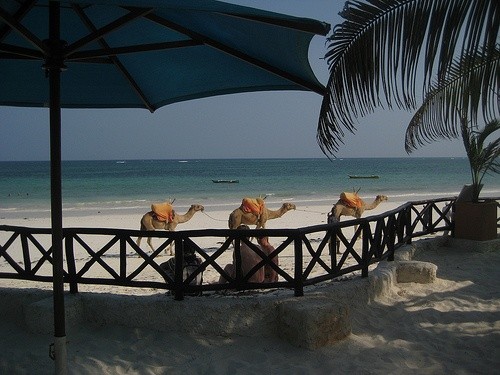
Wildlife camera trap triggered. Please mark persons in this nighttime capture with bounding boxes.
[328,208,342,254]
[218,224,277,283]
[161,240,202,295]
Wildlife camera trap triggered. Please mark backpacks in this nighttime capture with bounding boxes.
[182,260,204,296]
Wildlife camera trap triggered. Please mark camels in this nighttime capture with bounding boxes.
[228,202,296,245]
[333,194,388,238]
[135,203,204,255]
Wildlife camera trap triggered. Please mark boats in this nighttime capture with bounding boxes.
[210,180,240,184]
[346,175,378,179]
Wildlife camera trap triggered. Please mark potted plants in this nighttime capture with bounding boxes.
[454,111,500,241]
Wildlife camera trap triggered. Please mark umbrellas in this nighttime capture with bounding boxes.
[0,0,331,375]
